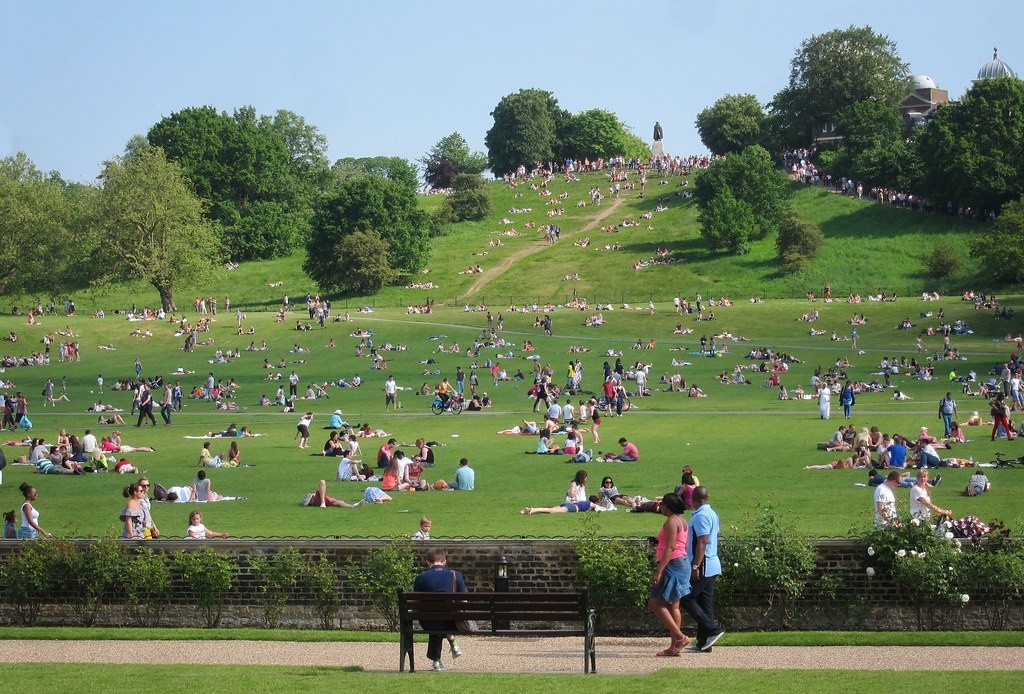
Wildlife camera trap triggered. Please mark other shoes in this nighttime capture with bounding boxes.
[149,447,157,452]
[74,468,86,475]
[966,482,975,496]
[934,473,941,486]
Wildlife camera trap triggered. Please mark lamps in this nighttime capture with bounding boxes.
[494,555,509,578]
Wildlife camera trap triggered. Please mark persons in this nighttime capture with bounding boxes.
[958,207,970,217]
[429,187,451,194]
[0,280,1024,540]
[646,493,690,656]
[414,548,465,670]
[784,148,930,212]
[653,122,663,141]
[990,210,995,224]
[225,261,239,271]
[680,485,724,652]
[457,154,726,281]
[948,200,952,215]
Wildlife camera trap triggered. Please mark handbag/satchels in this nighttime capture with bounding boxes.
[991,407,1005,415]
[18,414,32,428]
[450,569,478,632]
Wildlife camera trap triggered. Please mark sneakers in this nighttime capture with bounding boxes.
[701,631,724,651]
[684,645,703,653]
[433,660,445,672]
[452,647,462,660]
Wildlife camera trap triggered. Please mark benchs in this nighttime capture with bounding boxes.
[397,586,600,674]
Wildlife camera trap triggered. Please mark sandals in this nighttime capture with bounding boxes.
[657,648,681,656]
[671,635,690,655]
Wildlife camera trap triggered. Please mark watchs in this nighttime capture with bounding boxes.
[692,565,699,571]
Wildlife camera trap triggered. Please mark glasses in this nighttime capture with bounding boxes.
[137,490,143,493]
[140,485,150,488]
[860,445,866,447]
[605,481,612,484]
[416,459,421,463]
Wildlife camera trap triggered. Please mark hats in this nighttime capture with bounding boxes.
[334,410,342,415]
[920,426,929,431]
